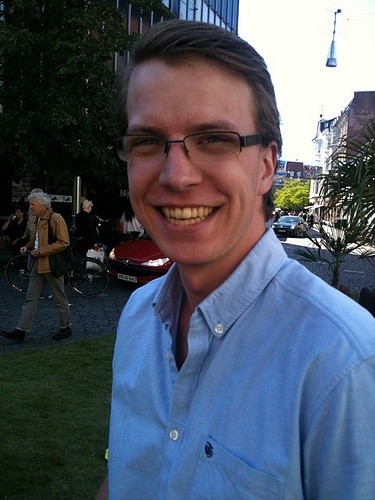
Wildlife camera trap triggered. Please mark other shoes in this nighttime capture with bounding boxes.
[51,327,72,341]
[0,329,25,342]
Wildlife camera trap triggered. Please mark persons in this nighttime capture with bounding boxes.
[1,207,28,275]
[13,188,46,280]
[0,192,72,342]
[96,18,375,499]
[71,199,100,268]
[119,202,145,239]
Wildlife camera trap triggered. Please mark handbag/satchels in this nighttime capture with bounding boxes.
[86,247,105,272]
[48,211,76,279]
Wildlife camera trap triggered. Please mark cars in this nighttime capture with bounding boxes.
[110,226,173,291]
[271,215,308,237]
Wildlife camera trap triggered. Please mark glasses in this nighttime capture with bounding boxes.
[116,131,265,163]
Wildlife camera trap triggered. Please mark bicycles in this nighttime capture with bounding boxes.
[5,253,108,297]
